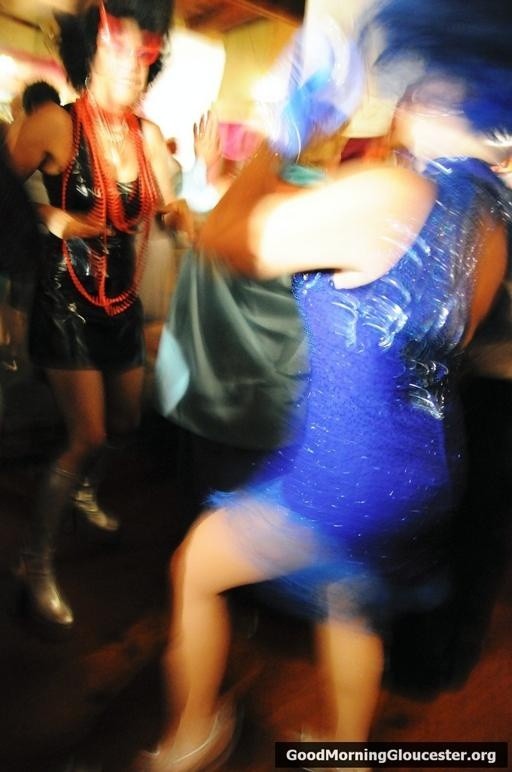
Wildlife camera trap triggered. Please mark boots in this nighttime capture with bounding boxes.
[70,431,128,534]
[13,465,77,630]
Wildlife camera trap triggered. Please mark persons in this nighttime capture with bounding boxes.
[0,0,512,772]
